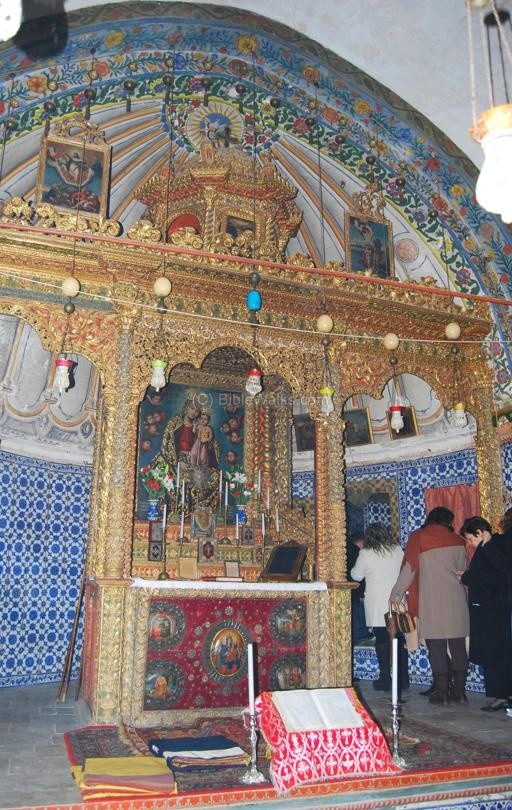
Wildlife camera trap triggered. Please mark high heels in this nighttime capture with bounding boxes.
[480,696,512,717]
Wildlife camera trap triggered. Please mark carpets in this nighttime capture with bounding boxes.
[63,715,512,809]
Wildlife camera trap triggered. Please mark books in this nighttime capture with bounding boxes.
[201,576,243,582]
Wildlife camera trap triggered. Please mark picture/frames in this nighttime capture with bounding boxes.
[35,135,111,221]
[224,559,241,578]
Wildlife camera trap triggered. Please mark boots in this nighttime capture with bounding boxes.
[418,670,468,706]
[398,649,409,689]
[373,643,390,691]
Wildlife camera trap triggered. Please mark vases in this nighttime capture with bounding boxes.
[146,499,160,522]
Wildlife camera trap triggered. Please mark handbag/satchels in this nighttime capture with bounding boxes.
[384,601,416,633]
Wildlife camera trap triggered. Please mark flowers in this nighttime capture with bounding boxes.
[140,462,175,500]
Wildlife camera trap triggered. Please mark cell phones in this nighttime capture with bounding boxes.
[450,569,463,577]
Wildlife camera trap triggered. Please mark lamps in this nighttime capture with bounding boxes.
[467,0,512,225]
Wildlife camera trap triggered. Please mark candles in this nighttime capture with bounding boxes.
[392,637,398,705]
[248,642,254,716]
[162,462,279,539]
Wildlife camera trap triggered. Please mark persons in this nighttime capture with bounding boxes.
[384,507,470,707]
[350,524,411,689]
[191,405,214,464]
[346,532,365,642]
[63,150,85,182]
[161,391,220,469]
[48,183,99,213]
[448,515,511,711]
